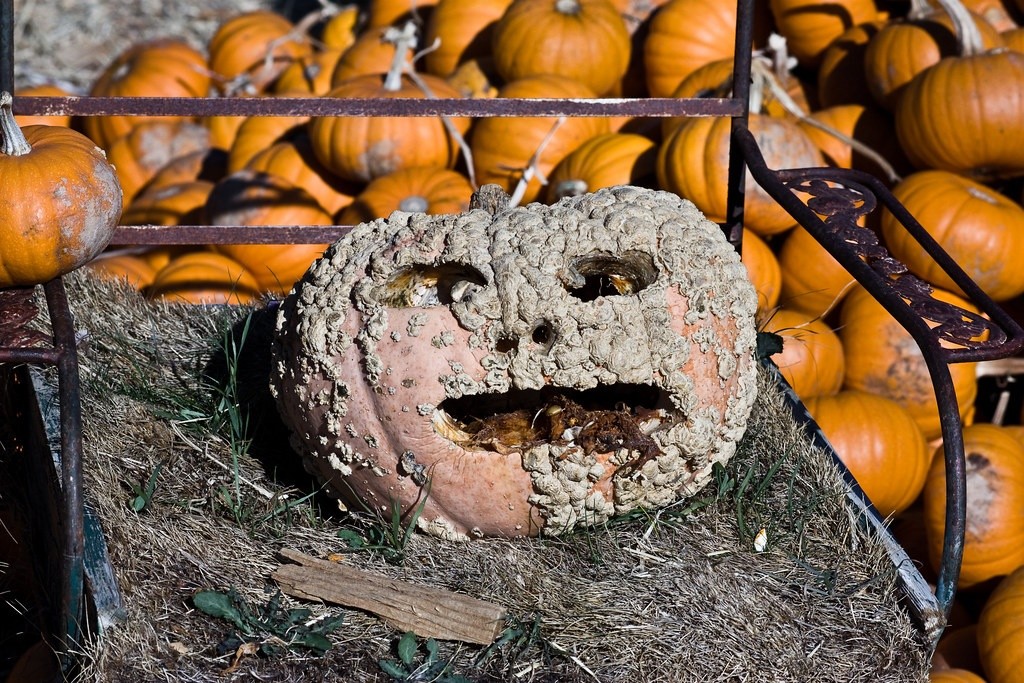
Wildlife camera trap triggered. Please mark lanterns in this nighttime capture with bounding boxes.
[262,182,760,541]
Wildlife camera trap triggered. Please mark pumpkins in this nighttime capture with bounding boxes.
[0,0,1024,683]
